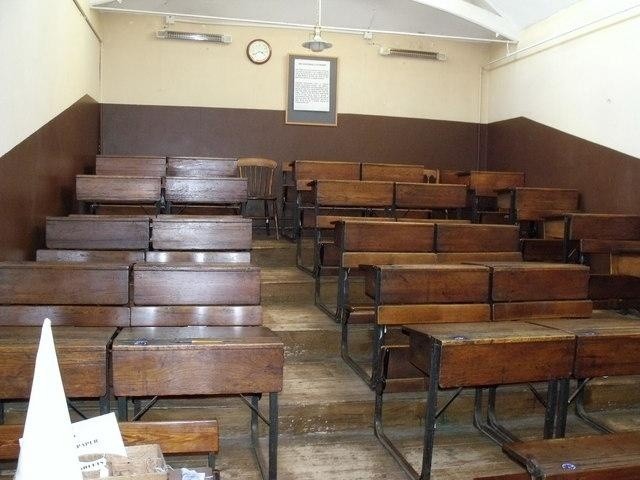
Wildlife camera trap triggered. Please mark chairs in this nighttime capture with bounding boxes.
[236,156,283,243]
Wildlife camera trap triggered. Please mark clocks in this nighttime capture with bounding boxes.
[247,38,272,64]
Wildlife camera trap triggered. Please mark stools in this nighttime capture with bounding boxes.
[0,154,290,477]
[289,156,637,471]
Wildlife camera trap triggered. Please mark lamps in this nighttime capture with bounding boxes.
[302,1,333,52]
